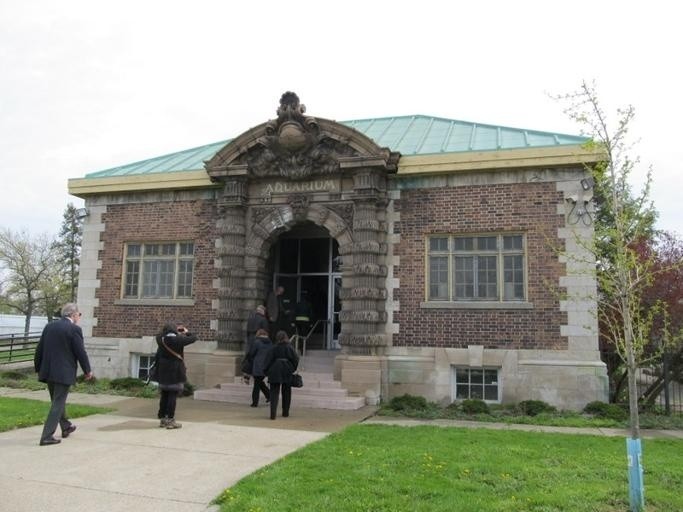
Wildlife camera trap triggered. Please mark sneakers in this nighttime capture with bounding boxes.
[250,397,271,407]
[166,419,181,429]
[271,412,288,419]
[160,418,166,426]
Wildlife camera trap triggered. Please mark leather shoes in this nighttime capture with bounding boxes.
[62,426,75,437]
[40,439,58,444]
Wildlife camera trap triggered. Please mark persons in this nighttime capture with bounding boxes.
[154,322,198,430]
[290,290,315,358]
[261,329,302,422]
[244,328,275,408]
[264,285,288,346]
[33,303,94,446]
[245,304,273,350]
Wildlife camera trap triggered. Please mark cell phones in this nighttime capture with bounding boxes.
[178,329,184,332]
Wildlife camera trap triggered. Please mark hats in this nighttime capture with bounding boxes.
[163,323,178,336]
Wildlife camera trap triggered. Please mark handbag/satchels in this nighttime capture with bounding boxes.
[148,359,159,381]
[291,375,302,387]
[240,356,253,373]
